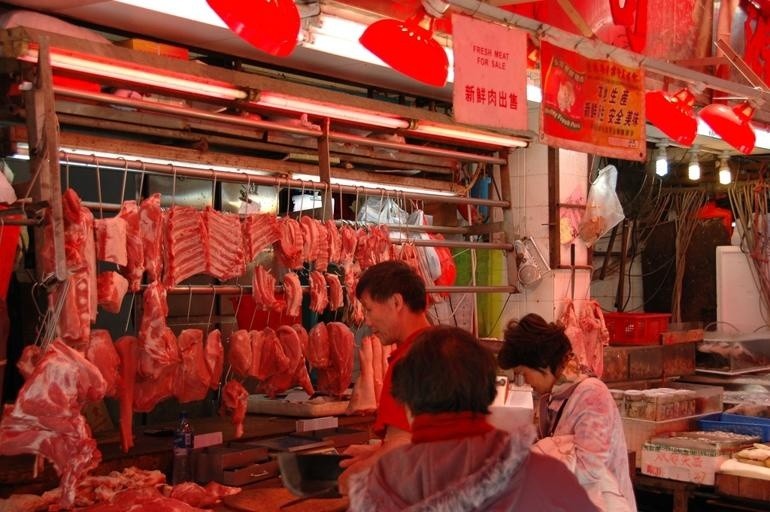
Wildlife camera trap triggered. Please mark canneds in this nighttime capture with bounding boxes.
[608,388,696,421]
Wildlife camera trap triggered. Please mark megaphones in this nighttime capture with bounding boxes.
[502,237,551,288]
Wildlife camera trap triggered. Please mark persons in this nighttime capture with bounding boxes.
[493,310,640,511]
[337,258,437,502]
[345,322,602,512]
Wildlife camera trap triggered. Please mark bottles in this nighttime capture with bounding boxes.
[171,411,195,485]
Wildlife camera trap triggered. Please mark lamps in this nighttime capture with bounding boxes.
[644,77,767,185]
[207,1,450,88]
